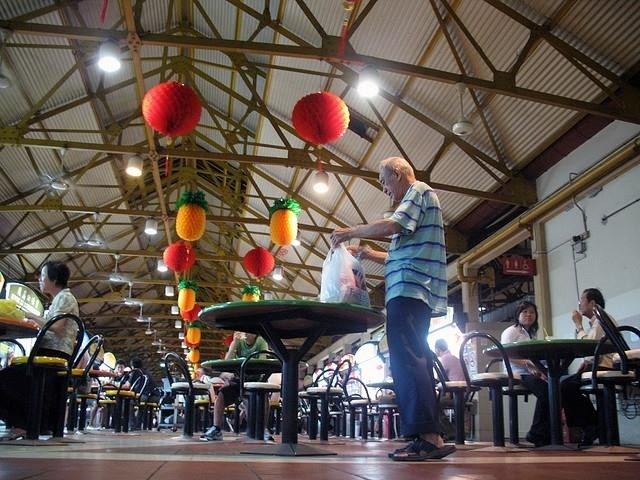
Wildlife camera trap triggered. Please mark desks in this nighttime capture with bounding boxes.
[481,338,617,454]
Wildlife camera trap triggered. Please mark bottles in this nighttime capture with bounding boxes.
[354,250,363,262]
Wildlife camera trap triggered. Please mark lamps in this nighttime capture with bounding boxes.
[312,170,328,195]
[125,152,145,177]
[293,230,301,248]
[156,256,197,379]
[98,37,122,74]
[356,63,380,98]
[272,263,284,281]
[144,217,159,236]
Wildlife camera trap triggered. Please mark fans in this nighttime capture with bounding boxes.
[0,27,17,89]
[37,147,71,190]
[78,212,169,361]
[452,82,473,137]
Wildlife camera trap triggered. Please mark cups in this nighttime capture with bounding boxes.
[112,381,120,390]
[545,336,556,341]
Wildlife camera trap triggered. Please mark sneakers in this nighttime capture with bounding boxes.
[525,433,551,446]
[577,427,598,445]
[1,428,26,440]
[87,426,99,431]
[263,430,276,443]
[199,426,223,441]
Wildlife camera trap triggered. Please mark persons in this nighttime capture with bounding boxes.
[558,287,627,451]
[435,340,467,403]
[329,157,456,461]
[200,328,276,442]
[67,333,177,437]
[0,262,79,442]
[501,301,560,448]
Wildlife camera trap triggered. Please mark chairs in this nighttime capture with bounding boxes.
[1,300,402,442]
[428,349,480,445]
[459,334,535,450]
[485,357,528,443]
[591,304,639,374]
[580,326,640,453]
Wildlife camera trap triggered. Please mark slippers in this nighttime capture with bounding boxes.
[388,439,456,461]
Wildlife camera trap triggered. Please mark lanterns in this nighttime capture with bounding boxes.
[162,240,197,273]
[175,190,207,260]
[242,247,274,279]
[270,196,301,246]
[143,80,202,178]
[177,281,261,378]
[292,93,350,175]
